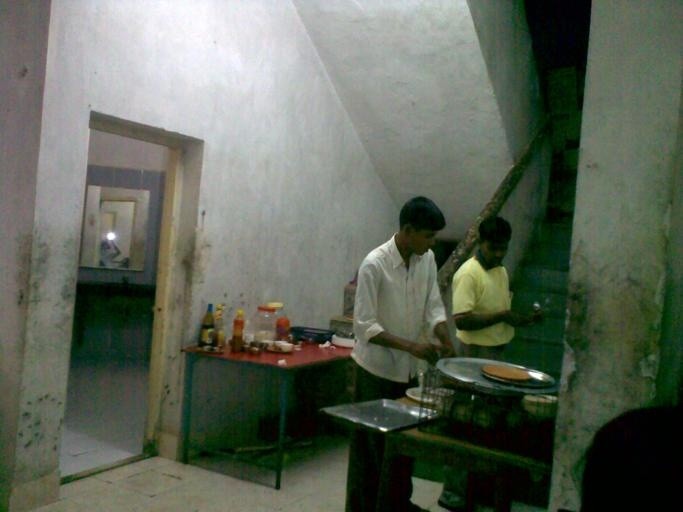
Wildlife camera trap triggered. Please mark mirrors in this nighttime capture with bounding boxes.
[78,183,151,273]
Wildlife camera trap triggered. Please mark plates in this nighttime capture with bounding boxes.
[405,384,446,406]
[479,364,533,382]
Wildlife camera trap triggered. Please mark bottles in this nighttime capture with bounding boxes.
[196,301,290,354]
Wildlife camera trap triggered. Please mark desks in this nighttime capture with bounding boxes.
[334,397,552,512]
[180,342,354,489]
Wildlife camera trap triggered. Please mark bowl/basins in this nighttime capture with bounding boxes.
[522,394,559,419]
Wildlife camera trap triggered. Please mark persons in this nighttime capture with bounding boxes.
[438,216,536,512]
[343,195,454,512]
[100,239,121,266]
